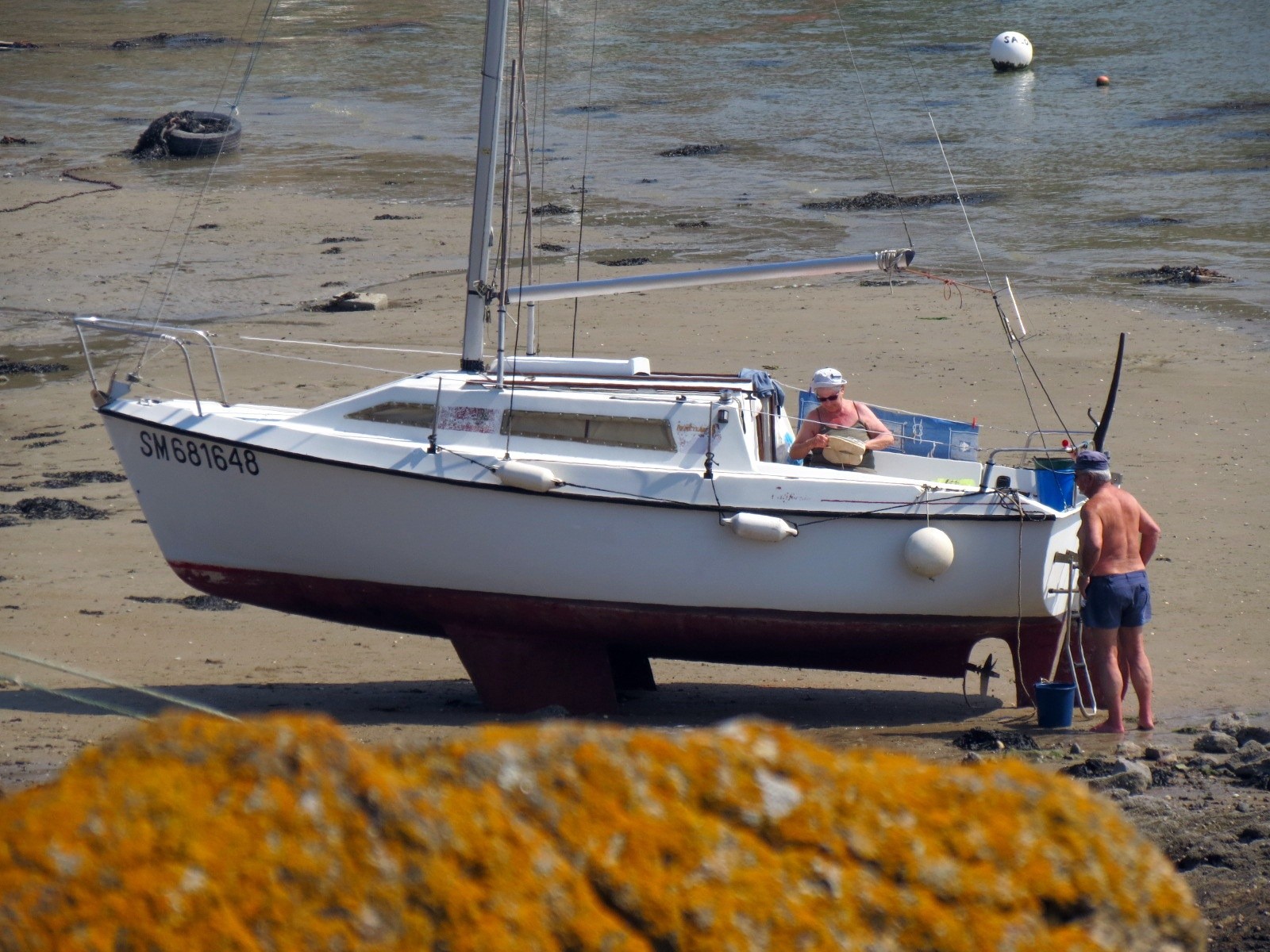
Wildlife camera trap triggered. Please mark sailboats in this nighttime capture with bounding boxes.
[75,0,1131,716]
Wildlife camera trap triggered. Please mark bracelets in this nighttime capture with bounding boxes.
[805,438,815,451]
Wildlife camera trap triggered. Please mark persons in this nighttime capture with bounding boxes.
[789,368,894,474]
[1075,451,1161,734]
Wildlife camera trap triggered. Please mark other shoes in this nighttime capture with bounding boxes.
[822,447,863,466]
[826,434,866,455]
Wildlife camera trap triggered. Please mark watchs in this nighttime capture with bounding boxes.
[1079,571,1087,578]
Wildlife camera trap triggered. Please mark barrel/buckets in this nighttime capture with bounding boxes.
[1032,456,1076,475]
[1034,469,1076,511]
[1034,680,1077,729]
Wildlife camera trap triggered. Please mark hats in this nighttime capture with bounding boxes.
[1071,451,1109,470]
[809,368,847,388]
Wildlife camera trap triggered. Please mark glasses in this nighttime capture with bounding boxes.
[816,389,841,403]
